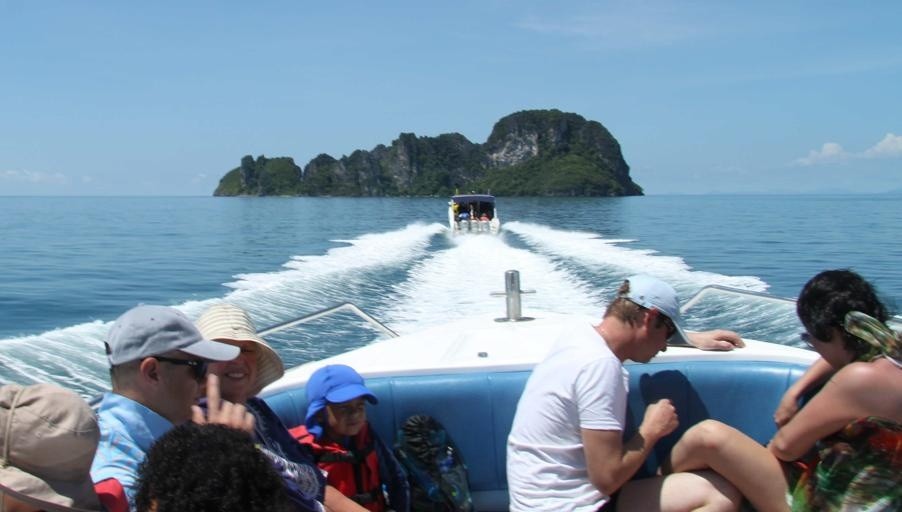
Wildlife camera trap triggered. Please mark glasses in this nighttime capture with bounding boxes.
[153,354,209,383]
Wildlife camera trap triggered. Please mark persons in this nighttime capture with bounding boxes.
[0,307,394,511]
[506,275,739,511]
[452,202,490,222]
[659,270,902,512]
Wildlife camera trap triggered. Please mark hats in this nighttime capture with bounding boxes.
[193,304,285,395]
[302,363,378,426]
[615,275,696,349]
[0,384,100,512]
[105,304,241,364]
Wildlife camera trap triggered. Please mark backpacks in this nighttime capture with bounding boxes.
[393,413,473,510]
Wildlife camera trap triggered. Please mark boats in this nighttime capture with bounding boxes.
[447,188,500,237]
[76,285,902,510]
